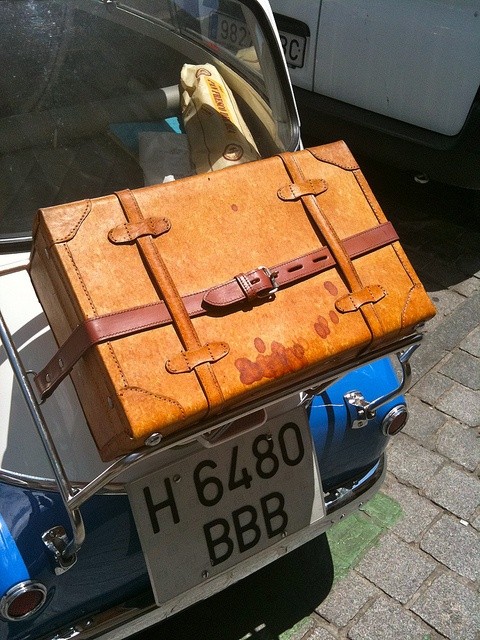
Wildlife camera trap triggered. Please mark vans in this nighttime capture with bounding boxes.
[2,0,421,638]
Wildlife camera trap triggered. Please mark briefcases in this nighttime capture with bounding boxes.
[26,139,436,462]
